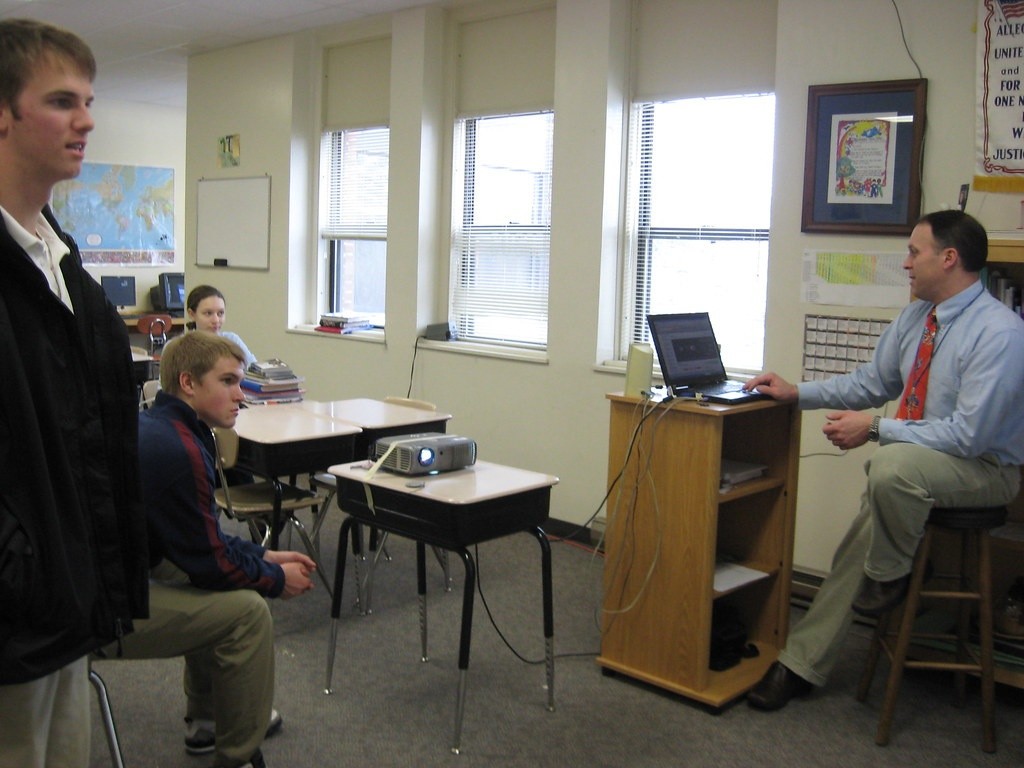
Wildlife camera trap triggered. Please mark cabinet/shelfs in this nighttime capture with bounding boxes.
[957,242,1024,707]
[600,388,809,707]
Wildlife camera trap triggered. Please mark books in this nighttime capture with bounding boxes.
[314,312,373,334]
[978,267,1024,320]
[240,359,306,407]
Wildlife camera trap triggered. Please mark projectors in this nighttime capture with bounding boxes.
[374,432,477,475]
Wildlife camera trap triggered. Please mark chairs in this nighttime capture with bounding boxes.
[130,345,148,354]
[206,423,335,613]
[137,314,173,378]
[143,378,162,406]
[376,399,434,575]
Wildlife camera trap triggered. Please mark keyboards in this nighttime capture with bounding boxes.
[120,315,141,320]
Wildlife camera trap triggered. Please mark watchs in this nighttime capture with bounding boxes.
[867,416,881,443]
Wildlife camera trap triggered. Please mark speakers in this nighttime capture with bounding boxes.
[624,343,654,399]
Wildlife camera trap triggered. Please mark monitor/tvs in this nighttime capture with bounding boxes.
[100,276,136,309]
[159,272,185,310]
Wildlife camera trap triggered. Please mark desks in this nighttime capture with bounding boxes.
[301,395,452,616]
[227,401,365,618]
[320,458,572,754]
[131,350,154,388]
[119,311,185,333]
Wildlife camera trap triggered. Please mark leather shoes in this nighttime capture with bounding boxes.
[852,572,912,620]
[746,660,821,712]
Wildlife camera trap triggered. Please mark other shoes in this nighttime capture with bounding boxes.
[184,708,282,753]
[213,747,266,768]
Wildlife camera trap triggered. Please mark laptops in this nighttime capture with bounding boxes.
[648,312,773,405]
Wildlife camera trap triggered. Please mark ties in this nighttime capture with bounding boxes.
[893,304,939,421]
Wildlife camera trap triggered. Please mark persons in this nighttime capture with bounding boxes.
[741,211,1024,712]
[0,19,148,768]
[186,285,257,366]
[88,330,317,768]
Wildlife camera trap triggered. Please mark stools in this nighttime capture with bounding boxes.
[844,505,1007,755]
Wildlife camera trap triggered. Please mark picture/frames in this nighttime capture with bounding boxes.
[799,77,928,235]
